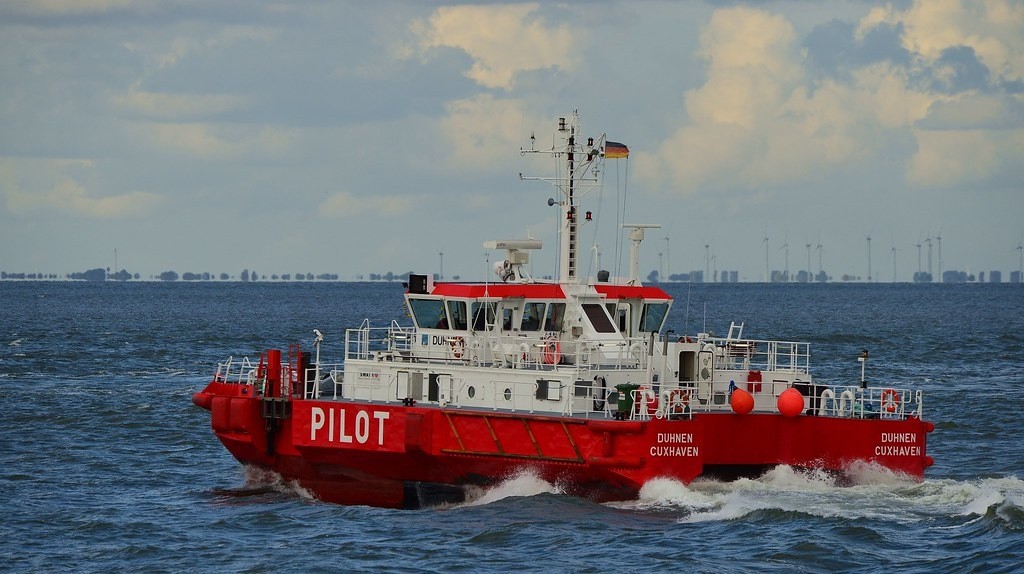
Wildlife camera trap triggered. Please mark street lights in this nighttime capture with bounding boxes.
[662,228,944,283]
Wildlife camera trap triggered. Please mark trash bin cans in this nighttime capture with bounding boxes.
[614,384,640,417]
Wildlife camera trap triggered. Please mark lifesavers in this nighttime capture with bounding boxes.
[452,336,464,358]
[669,389,687,411]
[678,337,693,343]
[883,390,899,412]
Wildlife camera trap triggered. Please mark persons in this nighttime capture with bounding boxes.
[436,306,450,329]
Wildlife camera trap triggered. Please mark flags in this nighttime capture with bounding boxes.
[604,140,630,158]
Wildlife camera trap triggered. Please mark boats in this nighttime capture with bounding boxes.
[193,108,936,507]
